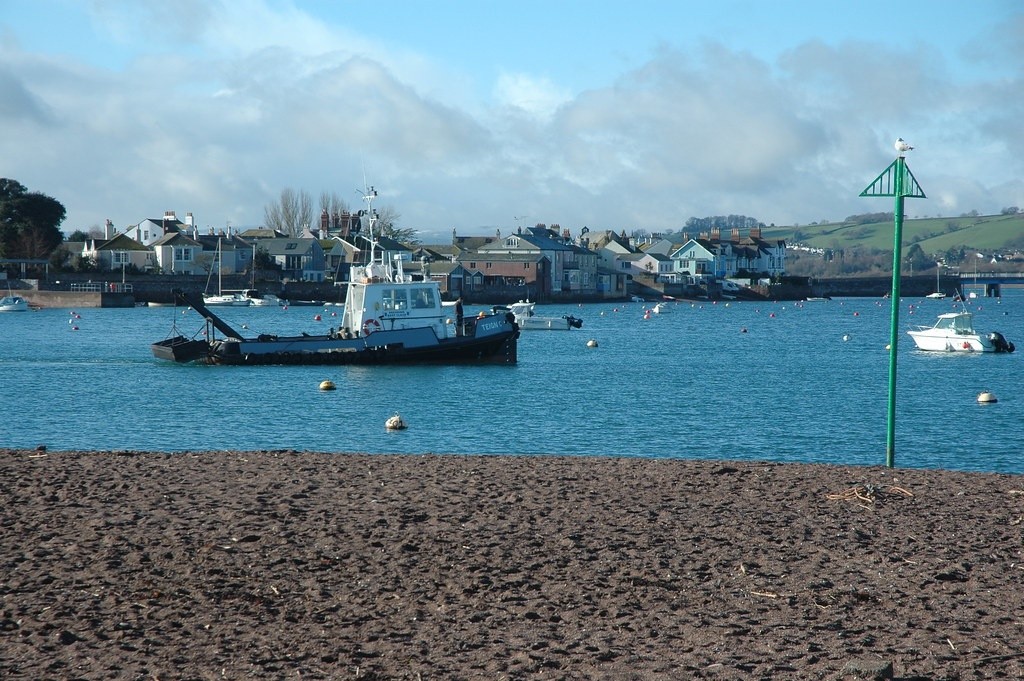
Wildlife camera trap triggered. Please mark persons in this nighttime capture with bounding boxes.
[454,297,464,337]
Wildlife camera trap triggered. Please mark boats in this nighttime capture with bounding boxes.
[806,296,830,302]
[0,295,29,311]
[925,292,947,300]
[906,287,1015,358]
[202,237,327,306]
[151,151,519,364]
[505,297,583,330]
